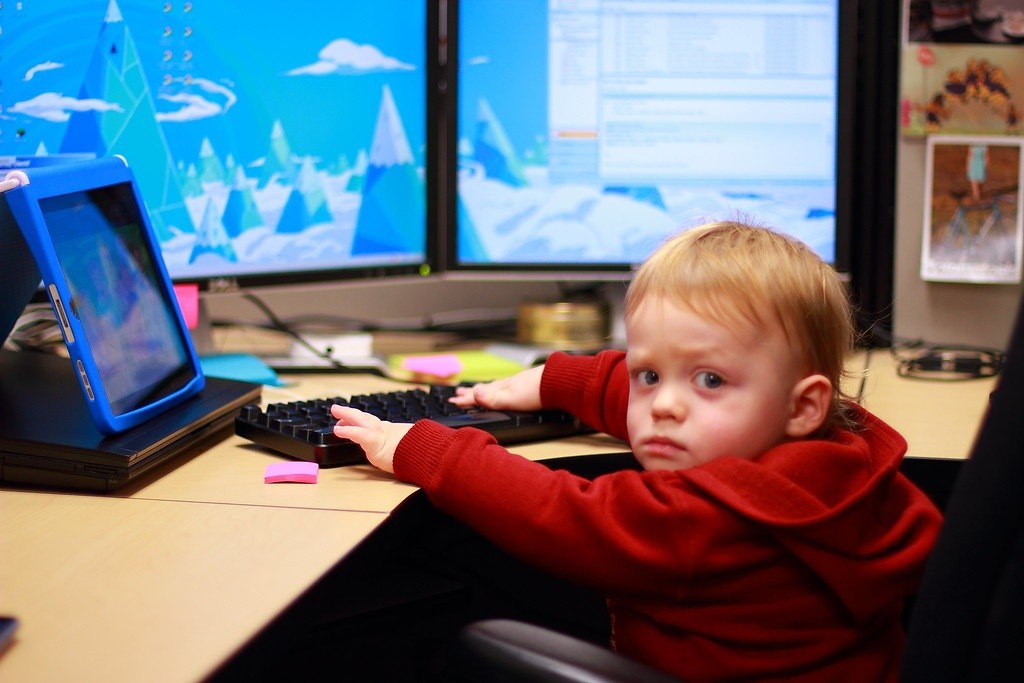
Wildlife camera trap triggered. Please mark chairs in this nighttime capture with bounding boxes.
[462,296,1024,683]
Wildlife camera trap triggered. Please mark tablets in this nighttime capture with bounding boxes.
[5,154,206,434]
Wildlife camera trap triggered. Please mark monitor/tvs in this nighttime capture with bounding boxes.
[0,0,857,357]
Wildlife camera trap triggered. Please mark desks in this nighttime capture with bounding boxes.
[0,491,390,683]
[858,350,1000,478]
[128,340,871,516]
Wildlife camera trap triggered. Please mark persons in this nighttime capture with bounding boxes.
[327,221,943,683]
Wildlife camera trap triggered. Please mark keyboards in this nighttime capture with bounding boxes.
[234,379,603,468]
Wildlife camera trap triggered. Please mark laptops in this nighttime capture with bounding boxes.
[0,349,262,481]
